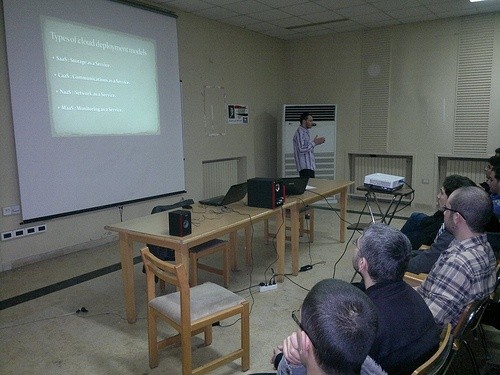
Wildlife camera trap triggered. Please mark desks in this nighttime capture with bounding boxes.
[104,178,355,324]
[357,185,416,225]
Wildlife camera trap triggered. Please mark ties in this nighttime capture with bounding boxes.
[434,222,445,244]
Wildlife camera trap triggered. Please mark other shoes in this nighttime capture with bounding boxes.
[305,214,310,219]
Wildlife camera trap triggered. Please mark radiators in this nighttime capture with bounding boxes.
[202,159,238,200]
[446,160,489,184]
[353,157,406,201]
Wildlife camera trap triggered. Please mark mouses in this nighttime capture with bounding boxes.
[182,205,192,209]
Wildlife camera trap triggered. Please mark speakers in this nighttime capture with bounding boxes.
[168,209,192,237]
[247,176,286,209]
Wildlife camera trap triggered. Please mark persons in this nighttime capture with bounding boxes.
[397,147,500,264]
[292,113,325,219]
[399,176,494,275]
[244,222,443,375]
[351,186,497,341]
[275,278,389,375]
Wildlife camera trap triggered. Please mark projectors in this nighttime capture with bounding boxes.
[364,173,405,188]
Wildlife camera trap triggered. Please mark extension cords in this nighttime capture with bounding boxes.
[256,283,277,292]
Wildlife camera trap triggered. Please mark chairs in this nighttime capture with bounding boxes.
[264,205,315,243]
[139,247,250,375]
[403,244,500,375]
[151,199,231,289]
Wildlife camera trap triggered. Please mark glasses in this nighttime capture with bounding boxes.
[291,310,318,351]
[442,206,466,220]
[352,238,364,257]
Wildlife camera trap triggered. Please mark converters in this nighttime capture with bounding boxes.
[300,265,313,271]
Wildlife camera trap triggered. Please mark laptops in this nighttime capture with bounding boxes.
[199,182,248,206]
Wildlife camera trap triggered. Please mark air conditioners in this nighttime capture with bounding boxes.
[276,103,338,205]
